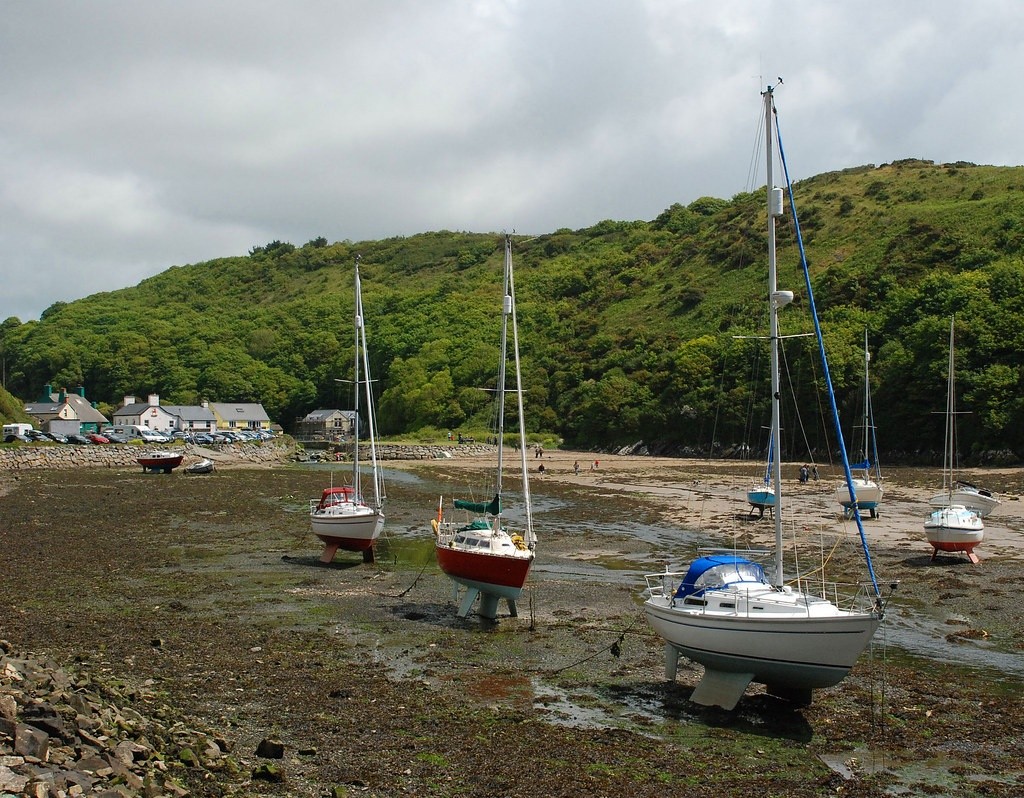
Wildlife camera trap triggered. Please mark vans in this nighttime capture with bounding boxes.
[114,424,156,441]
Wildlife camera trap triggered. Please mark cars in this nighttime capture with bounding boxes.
[151,429,277,444]
[5,430,128,445]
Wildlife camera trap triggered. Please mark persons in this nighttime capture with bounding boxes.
[539,463,545,475]
[573,462,580,474]
[595,460,598,467]
[514,443,518,453]
[493,436,498,447]
[447,431,455,442]
[535,446,543,459]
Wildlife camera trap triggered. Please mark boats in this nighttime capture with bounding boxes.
[136,455,184,468]
[186,460,216,473]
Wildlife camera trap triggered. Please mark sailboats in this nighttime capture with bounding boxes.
[921,316,1003,566]
[430,225,538,620]
[310,252,389,565]
[644,75,896,712]
[834,328,885,520]
[745,373,788,517]
